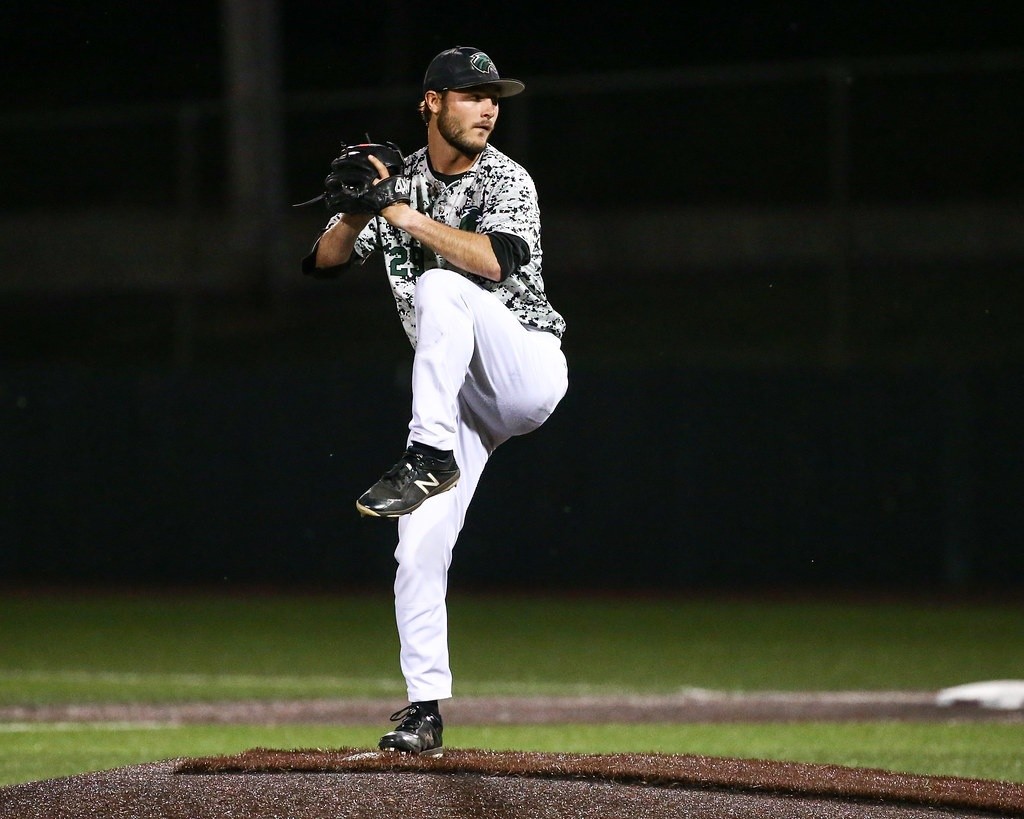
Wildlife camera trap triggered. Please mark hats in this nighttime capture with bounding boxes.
[424,45,525,97]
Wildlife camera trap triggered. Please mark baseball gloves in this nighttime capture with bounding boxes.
[290,132,412,217]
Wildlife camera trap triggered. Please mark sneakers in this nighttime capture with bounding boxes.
[379,704,442,760]
[355,447,460,517]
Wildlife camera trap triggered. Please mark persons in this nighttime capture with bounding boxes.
[301,42,568,756]
